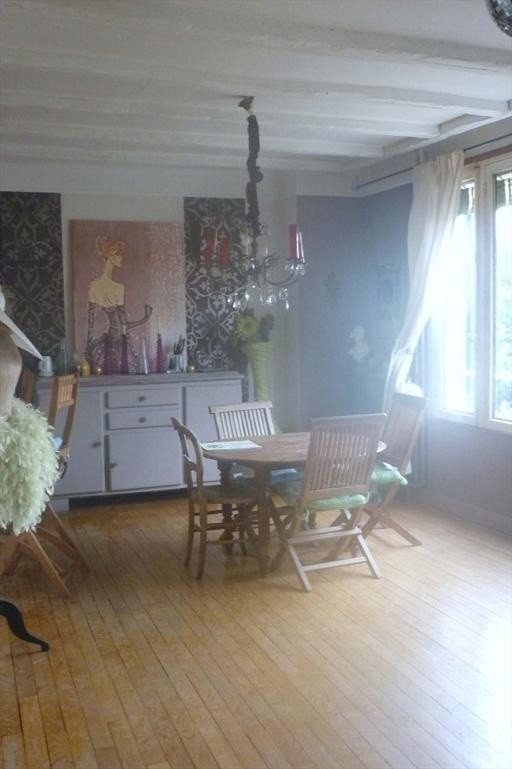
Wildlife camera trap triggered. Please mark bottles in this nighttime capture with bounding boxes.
[137,338,149,374]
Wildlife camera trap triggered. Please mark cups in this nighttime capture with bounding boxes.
[36,355,55,377]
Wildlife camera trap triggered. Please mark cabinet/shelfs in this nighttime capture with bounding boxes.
[31,369,244,516]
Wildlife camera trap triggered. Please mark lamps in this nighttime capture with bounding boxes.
[205,97,308,313]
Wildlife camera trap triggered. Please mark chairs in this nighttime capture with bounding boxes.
[0,373,91,600]
[172,392,428,592]
